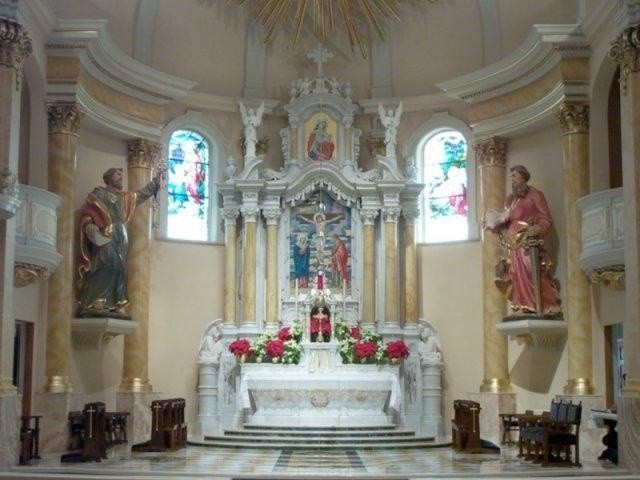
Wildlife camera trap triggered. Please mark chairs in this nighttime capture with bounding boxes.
[498,397,583,470]
[20,410,131,461]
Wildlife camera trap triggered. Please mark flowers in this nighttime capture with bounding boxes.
[227,317,412,372]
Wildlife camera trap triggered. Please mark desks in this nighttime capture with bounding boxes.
[584,407,618,467]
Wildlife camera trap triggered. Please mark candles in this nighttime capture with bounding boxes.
[295,277,299,317]
[343,277,346,317]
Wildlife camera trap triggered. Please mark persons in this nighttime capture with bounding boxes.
[199,326,223,361]
[77,164,165,317]
[294,76,353,99]
[381,108,401,150]
[294,233,312,286]
[243,108,260,152]
[308,117,334,162]
[419,327,442,362]
[329,235,350,290]
[298,211,341,270]
[481,166,560,311]
